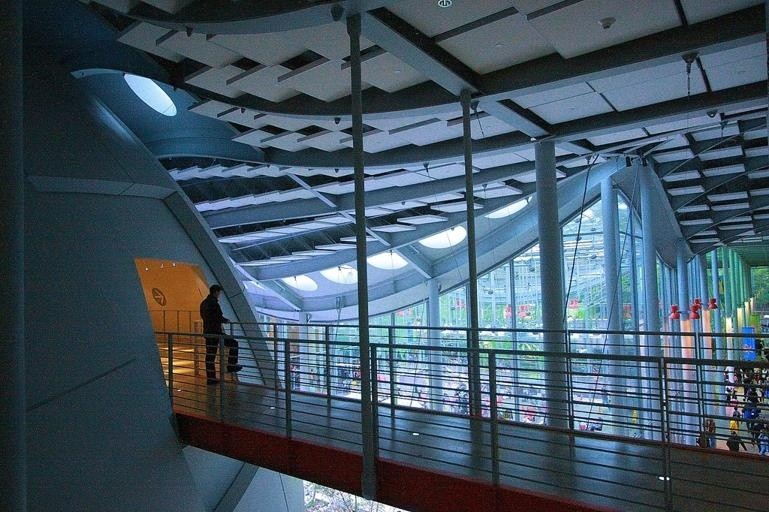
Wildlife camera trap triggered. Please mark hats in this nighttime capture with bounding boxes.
[210,285,223,292]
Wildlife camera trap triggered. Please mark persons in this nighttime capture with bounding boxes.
[288,364,610,431]
[200,286,243,384]
[694,366,769,455]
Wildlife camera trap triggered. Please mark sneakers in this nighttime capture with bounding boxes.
[207,379,220,384]
[227,366,242,372]
